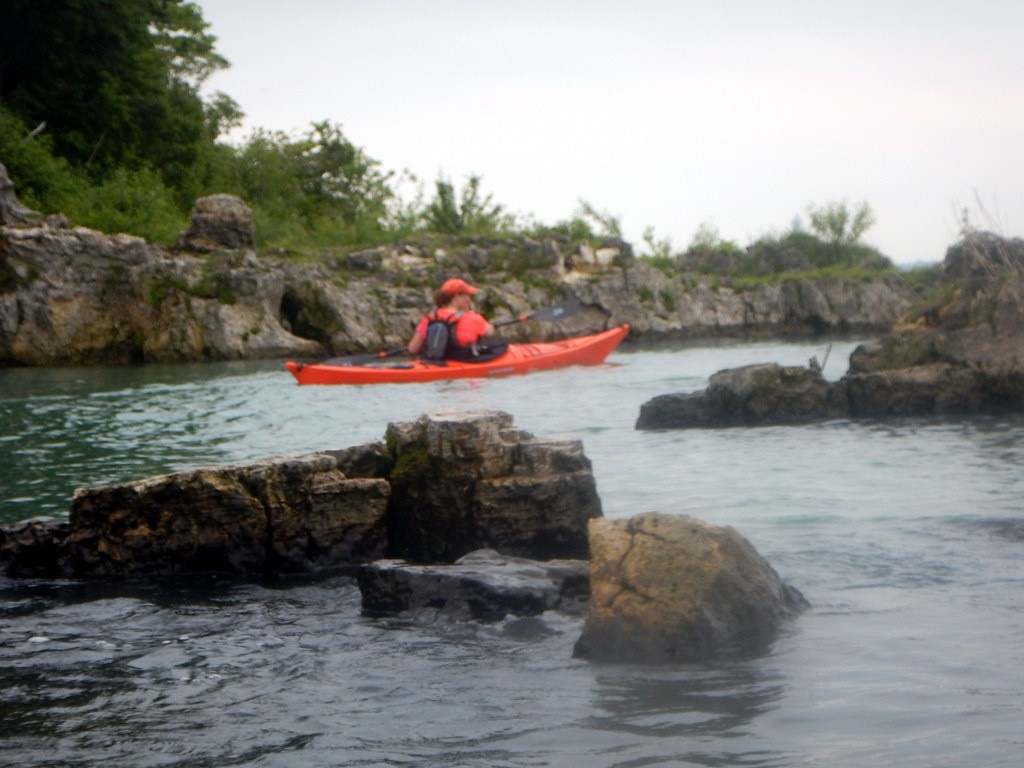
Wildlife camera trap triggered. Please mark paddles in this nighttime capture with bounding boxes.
[323,298,582,367]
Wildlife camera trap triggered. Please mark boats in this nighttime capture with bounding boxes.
[283,324,632,384]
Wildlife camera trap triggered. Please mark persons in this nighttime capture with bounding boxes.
[408,278,498,360]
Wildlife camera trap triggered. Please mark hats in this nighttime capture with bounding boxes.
[441,278,477,294]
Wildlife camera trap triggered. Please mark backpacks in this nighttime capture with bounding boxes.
[423,307,464,360]
